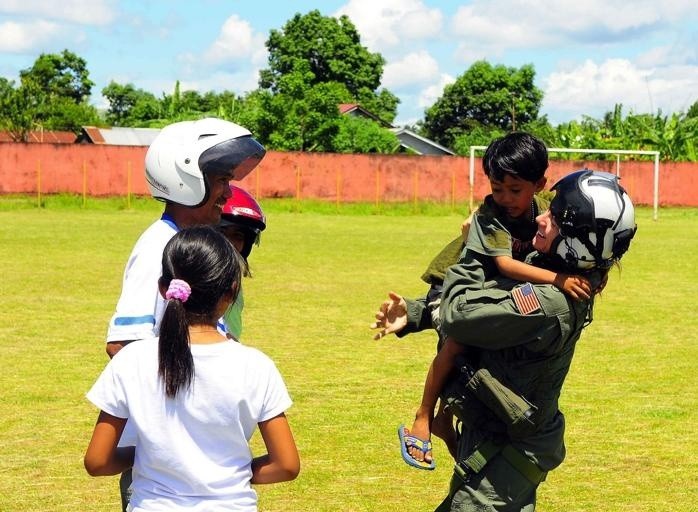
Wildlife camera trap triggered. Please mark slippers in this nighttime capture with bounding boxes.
[398,424,436,470]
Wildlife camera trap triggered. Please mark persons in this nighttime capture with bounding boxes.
[397,131,592,472]
[105,115,268,511]
[371,170,637,512]
[214,183,267,340]
[83,224,300,511]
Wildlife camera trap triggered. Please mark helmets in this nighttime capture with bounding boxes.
[145,117,266,235]
[549,169,637,272]
